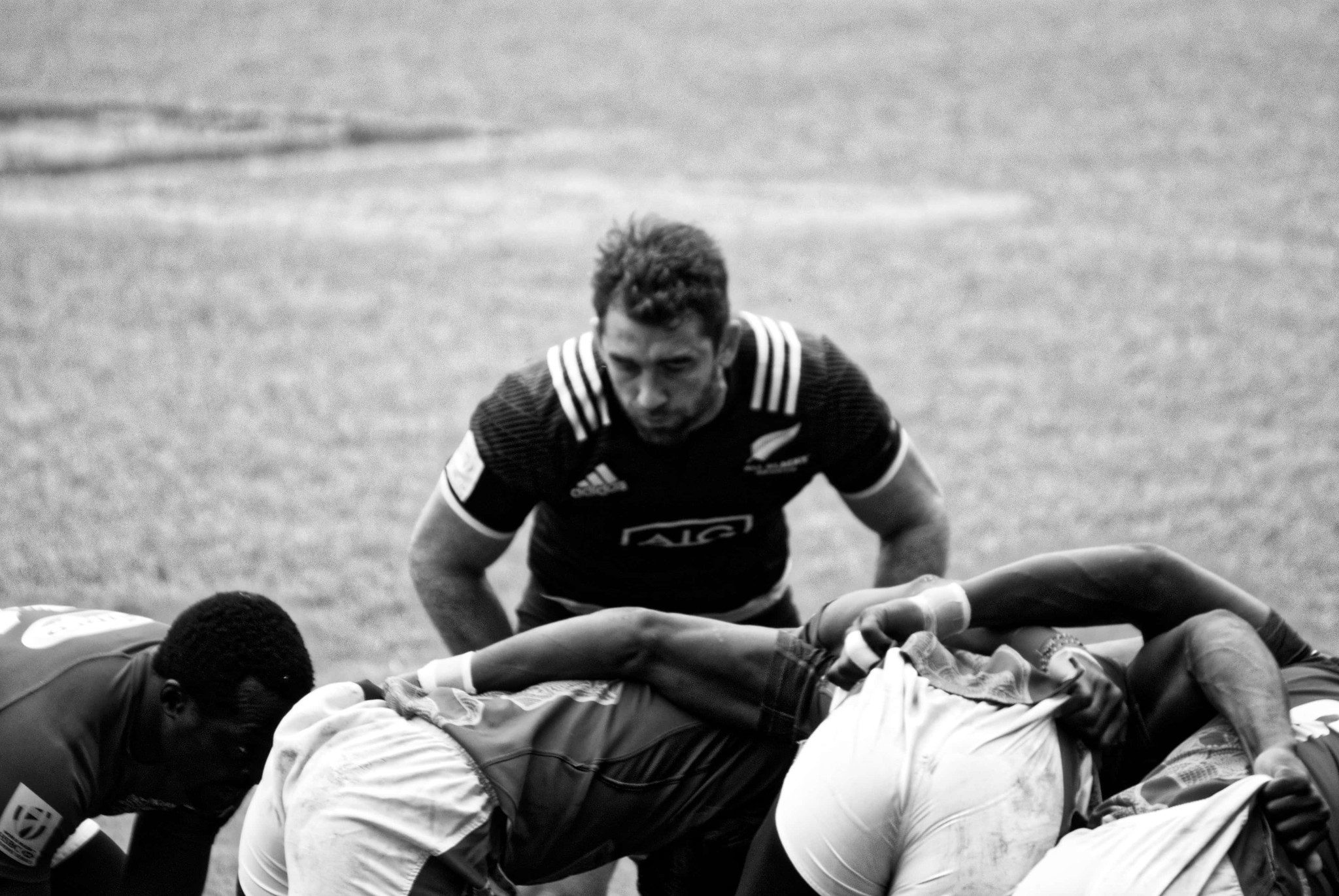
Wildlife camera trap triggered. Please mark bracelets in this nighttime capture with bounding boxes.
[911,581,971,639]
[416,650,476,695]
[1039,633,1083,672]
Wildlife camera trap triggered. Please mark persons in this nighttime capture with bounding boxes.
[407,216,951,896]
[239,544,1339,896]
[0,590,315,896]
